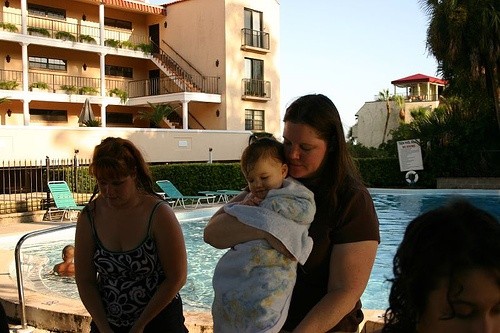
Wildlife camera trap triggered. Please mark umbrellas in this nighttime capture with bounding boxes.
[78,98,95,124]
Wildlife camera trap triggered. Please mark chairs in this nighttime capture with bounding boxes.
[46,181,85,221]
[156,177,244,210]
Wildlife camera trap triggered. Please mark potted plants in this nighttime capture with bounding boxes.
[0,80,129,103]
[0,21,152,56]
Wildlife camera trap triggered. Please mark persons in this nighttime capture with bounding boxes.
[203,93,381,333]
[74,136,188,333]
[382,198,500,332]
[212,138,314,332]
[53,245,77,273]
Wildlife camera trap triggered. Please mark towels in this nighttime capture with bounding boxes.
[211,178,316,333]
